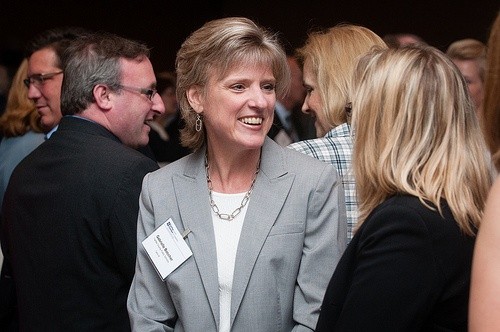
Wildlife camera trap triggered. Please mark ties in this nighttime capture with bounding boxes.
[286,116,296,140]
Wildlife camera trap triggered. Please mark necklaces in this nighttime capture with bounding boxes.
[203,150,261,220]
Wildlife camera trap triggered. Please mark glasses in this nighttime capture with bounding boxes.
[345,102,352,117]
[23,71,65,89]
[91,81,157,103]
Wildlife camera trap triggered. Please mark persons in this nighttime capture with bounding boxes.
[127,16,347,332]
[133,74,194,168]
[22,28,98,142]
[313,38,500,332]
[468,16,500,332]
[265,50,308,148]
[286,25,390,247]
[1,59,45,201]
[1,30,178,332]
[443,39,486,130]
